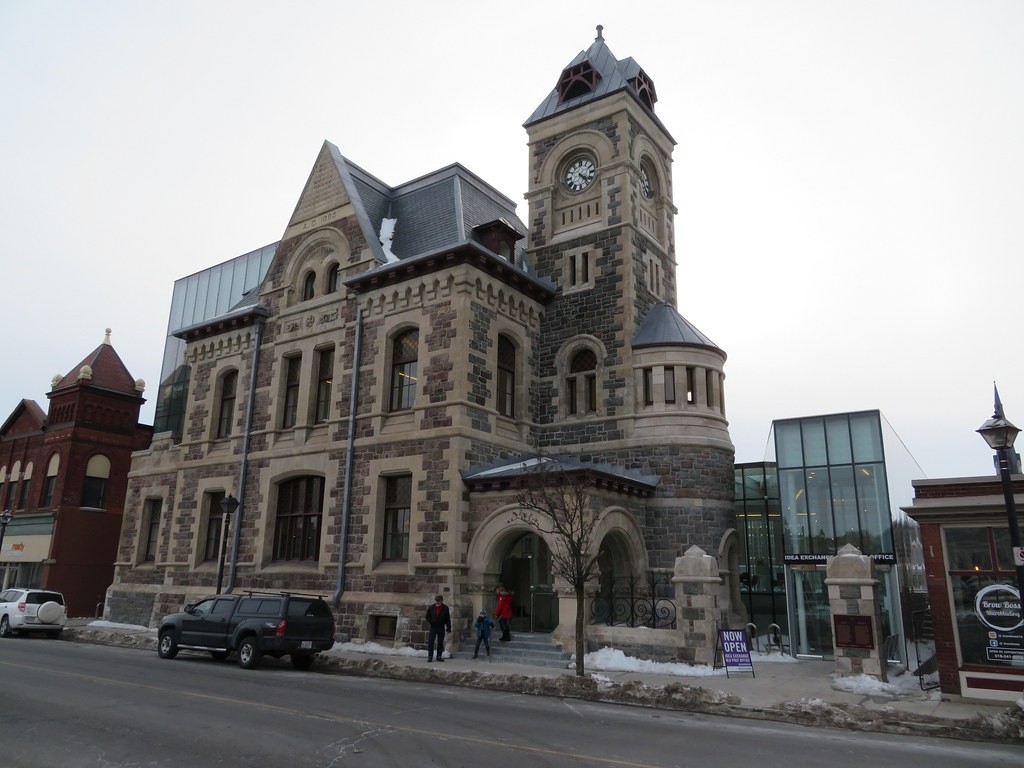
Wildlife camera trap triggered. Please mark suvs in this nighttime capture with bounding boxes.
[0,588,68,640]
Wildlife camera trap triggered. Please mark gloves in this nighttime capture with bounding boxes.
[489,622,494,629]
[446,626,451,633]
[478,617,482,622]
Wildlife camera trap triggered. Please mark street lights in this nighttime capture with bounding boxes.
[216,492,242,594]
[973,380,1024,622]
[0,508,16,552]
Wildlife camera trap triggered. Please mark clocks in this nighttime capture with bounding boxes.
[566,159,595,190]
[640,167,649,197]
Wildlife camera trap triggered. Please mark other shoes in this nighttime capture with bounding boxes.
[499,633,511,642]
[472,652,479,659]
[427,656,433,662]
[485,652,490,656]
[436,656,444,662]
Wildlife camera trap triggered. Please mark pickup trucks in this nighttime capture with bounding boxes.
[156,589,339,669]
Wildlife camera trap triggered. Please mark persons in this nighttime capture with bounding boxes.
[472,609,494,659]
[494,586,514,641]
[425,595,451,662]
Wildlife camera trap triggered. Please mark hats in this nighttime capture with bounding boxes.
[480,609,487,615]
[493,582,505,591]
[434,596,443,601]
